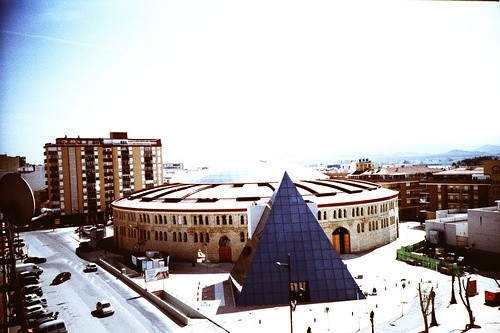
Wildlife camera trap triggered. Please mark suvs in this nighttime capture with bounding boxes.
[97,300,115,316]
[86,263,98,271]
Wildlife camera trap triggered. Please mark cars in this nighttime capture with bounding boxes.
[53,271,71,285]
[4,233,67,333]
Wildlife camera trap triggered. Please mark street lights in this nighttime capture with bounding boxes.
[276,253,294,333]
[431,291,438,326]
[450,269,457,304]
[370,311,374,333]
